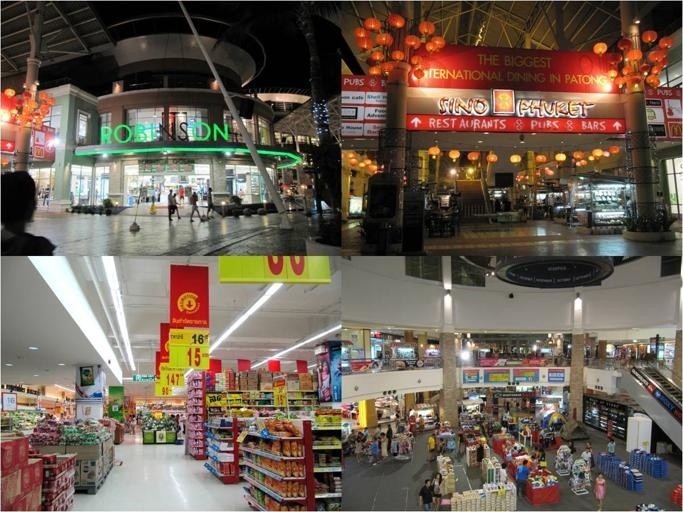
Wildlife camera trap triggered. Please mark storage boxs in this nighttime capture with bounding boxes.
[0,434,77,512]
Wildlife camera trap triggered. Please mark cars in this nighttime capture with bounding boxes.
[545,190,628,227]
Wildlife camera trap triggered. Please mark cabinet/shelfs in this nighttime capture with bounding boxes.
[583,395,628,441]
[0,407,42,436]
[32,436,114,494]
[113,429,124,444]
[142,427,177,444]
[185,370,343,512]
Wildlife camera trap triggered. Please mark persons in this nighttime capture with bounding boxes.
[347,410,616,511]
[167,185,215,222]
[42,184,49,205]
[1,170,57,256]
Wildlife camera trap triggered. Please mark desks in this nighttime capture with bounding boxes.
[518,431,533,447]
[493,434,560,506]
